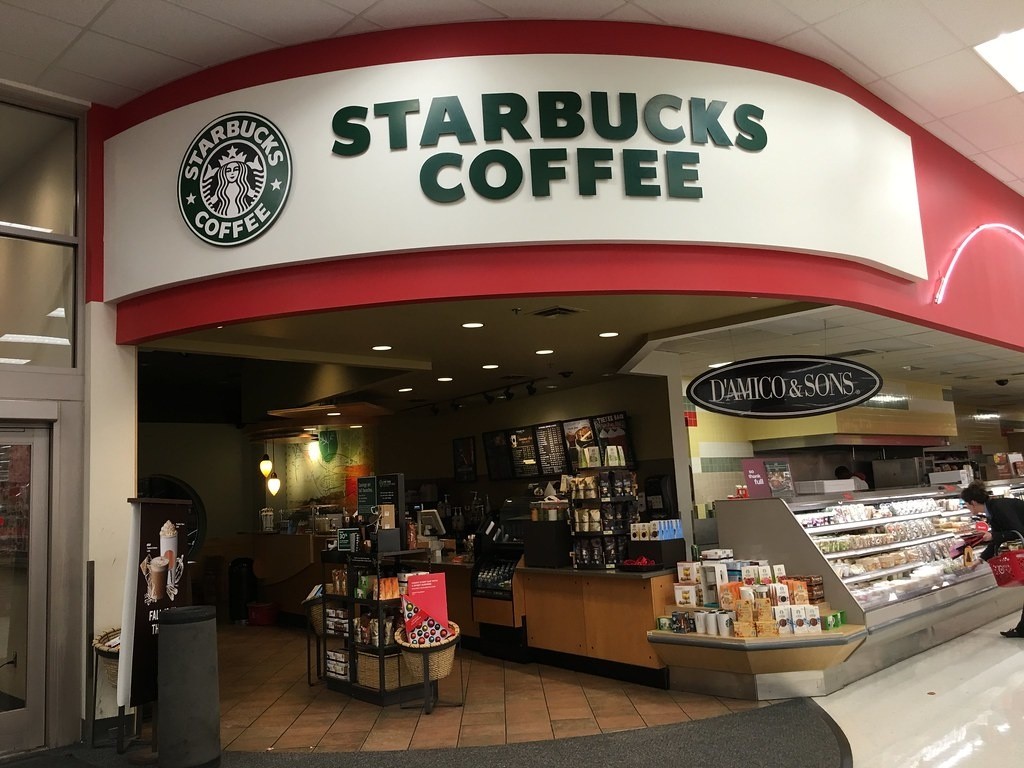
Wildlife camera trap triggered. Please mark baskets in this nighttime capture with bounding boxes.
[357,650,425,690]
[396,619,459,681]
[986,529,1024,588]
[92,626,121,689]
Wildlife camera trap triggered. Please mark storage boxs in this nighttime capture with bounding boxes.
[984,452,1012,481]
[656,545,849,638]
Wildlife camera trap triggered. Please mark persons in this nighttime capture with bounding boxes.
[835,466,869,490]
[961,481,1024,638]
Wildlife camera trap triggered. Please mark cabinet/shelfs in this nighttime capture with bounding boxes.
[715,476,1024,715]
[300,547,468,715]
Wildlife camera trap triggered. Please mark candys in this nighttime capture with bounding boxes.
[406,603,447,644]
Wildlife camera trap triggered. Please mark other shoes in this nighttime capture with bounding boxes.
[1000,629,1024,638]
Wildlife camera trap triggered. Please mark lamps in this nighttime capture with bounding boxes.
[260,430,281,497]
[429,381,537,417]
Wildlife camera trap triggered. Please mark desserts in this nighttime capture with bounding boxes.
[160,520,178,571]
